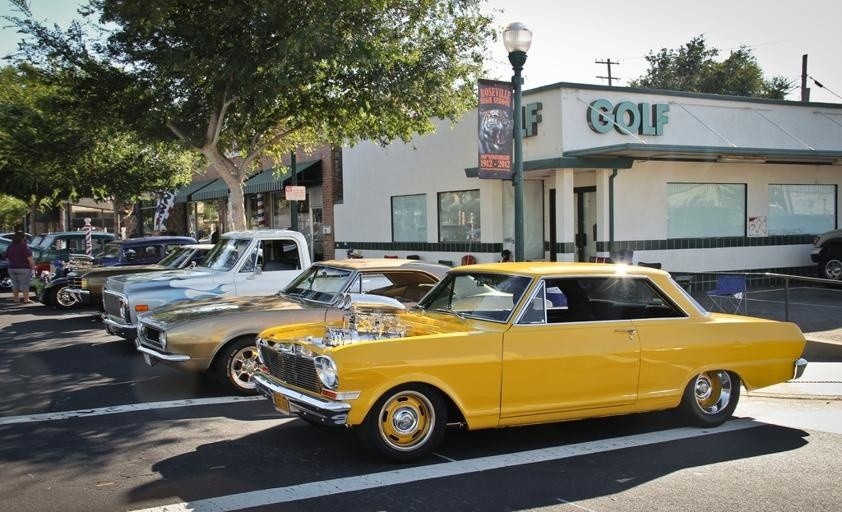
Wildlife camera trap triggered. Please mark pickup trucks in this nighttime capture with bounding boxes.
[98,228,395,341]
[26,216,265,311]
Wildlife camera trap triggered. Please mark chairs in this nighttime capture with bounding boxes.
[510,288,673,321]
[705,274,746,315]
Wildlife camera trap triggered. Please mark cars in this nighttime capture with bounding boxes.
[1,238,14,292]
[255,259,809,466]
[812,228,841,288]
[132,257,555,397]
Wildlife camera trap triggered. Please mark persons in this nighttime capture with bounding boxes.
[5,231,38,304]
[501,249,513,262]
[211,227,220,244]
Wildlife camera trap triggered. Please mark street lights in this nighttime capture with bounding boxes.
[500,21,534,264]
